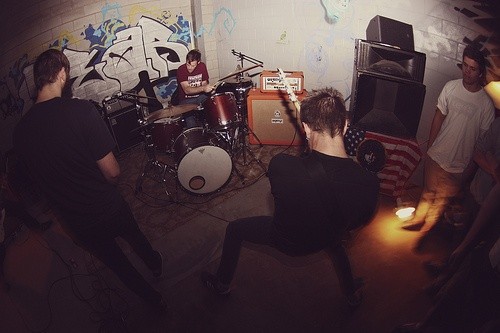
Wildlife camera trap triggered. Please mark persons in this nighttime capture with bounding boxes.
[177,49,216,128]
[399,50,500,333]
[201,87,379,312]
[13,49,164,298]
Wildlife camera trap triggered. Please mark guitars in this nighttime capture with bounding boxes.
[276,68,300,113]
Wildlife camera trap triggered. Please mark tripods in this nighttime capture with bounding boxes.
[133,98,179,203]
[229,86,270,178]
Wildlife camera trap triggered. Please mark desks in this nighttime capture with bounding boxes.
[344,123,420,201]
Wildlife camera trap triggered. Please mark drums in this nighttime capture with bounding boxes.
[172,128,234,195]
[201,91,243,130]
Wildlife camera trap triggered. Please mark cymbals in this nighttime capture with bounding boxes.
[144,104,198,121]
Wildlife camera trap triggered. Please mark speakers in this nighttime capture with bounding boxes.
[106,104,144,153]
[247,87,309,146]
[349,15,426,139]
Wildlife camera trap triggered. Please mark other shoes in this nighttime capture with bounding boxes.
[201,272,230,298]
[153,249,165,280]
[347,276,363,312]
